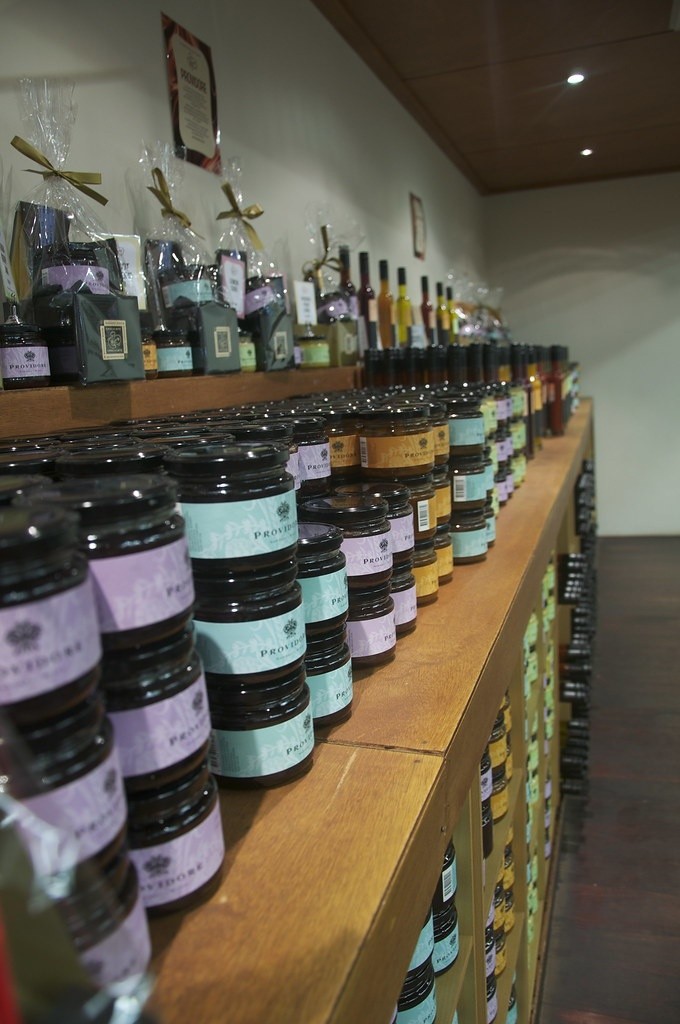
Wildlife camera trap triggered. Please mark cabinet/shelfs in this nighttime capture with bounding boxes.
[0,364,600,1023]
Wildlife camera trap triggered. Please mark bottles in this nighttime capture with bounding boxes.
[435,281,450,345]
[337,244,356,319]
[377,259,396,349]
[394,266,414,348]
[356,251,377,352]
[445,285,458,344]
[418,273,434,344]
